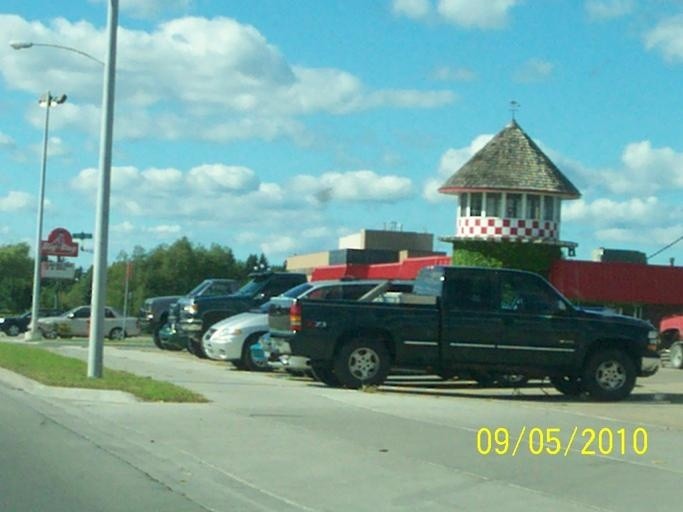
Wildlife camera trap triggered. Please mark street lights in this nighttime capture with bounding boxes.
[25,90,67,341]
[9,40,119,377]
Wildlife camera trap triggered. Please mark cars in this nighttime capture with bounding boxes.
[659,315,683,369]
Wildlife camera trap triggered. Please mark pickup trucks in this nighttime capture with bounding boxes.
[266,266,661,402]
